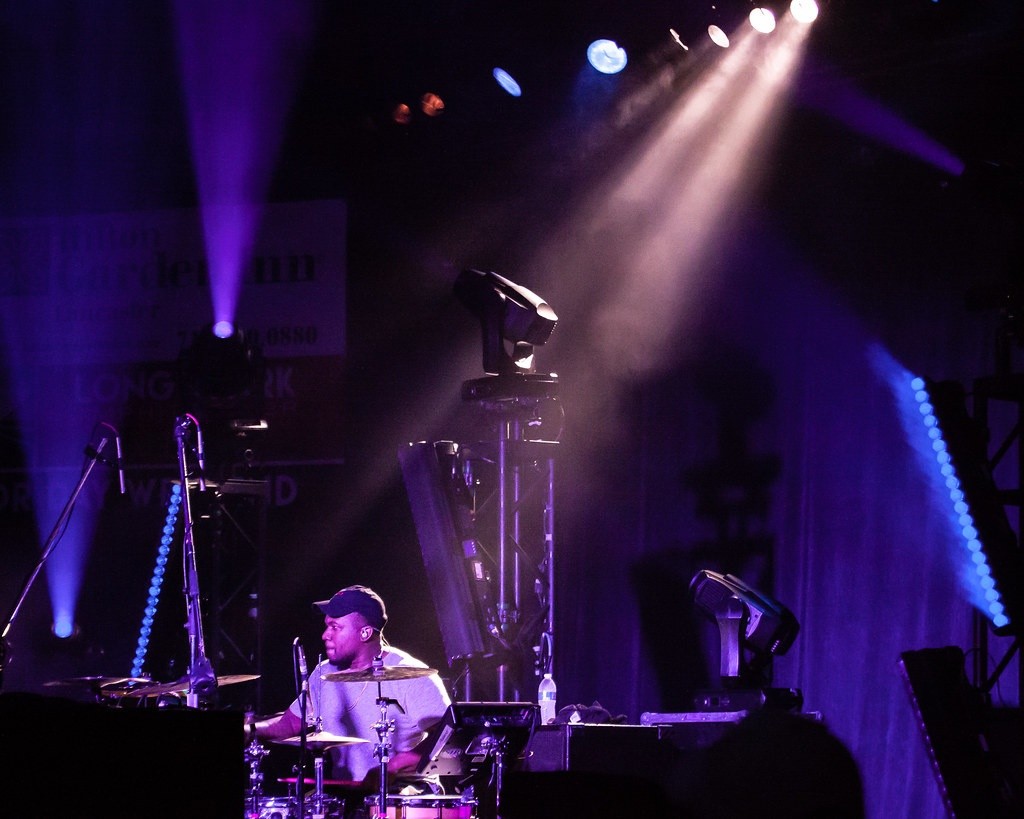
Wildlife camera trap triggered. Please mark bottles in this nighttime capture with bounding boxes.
[538,673,556,725]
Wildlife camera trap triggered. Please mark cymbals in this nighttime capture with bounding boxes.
[43,672,263,701]
[319,665,439,683]
[268,727,373,753]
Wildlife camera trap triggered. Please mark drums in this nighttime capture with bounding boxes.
[363,792,479,819]
[243,792,343,819]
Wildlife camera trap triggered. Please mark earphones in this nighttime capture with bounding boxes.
[362,632,368,638]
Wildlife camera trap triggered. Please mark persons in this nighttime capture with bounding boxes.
[244,585,463,819]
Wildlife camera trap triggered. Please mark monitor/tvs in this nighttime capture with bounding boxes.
[416,701,540,777]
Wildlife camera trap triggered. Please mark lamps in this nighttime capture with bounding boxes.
[670,0,831,51]
[688,569,801,711]
[452,268,559,401]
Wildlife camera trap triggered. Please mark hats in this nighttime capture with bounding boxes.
[313,586,387,628]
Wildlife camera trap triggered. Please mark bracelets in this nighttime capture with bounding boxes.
[250,722,256,733]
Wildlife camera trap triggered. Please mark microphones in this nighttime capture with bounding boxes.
[116,435,126,494]
[198,426,206,491]
[297,639,310,694]
